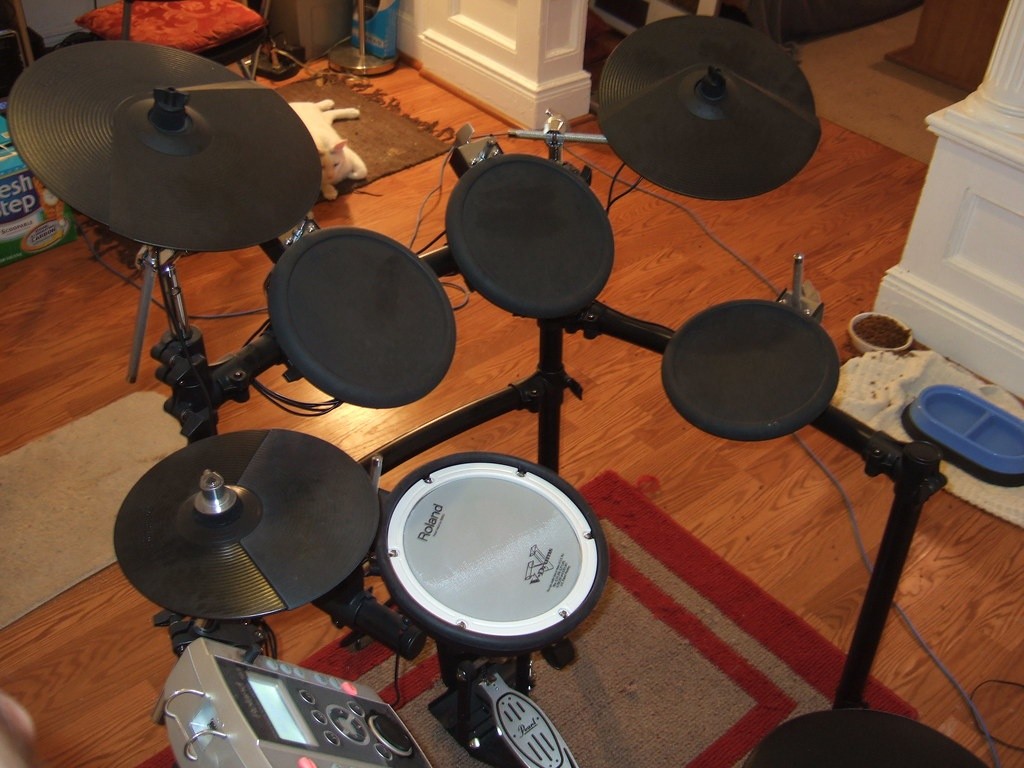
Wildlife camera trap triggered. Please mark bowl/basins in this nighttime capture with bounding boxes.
[849,313,914,356]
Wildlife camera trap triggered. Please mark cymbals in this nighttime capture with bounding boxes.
[112,426,378,617]
[597,13,820,200]
[7,38,323,253]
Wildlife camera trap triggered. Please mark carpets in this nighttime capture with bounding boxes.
[74,69,456,288]
[135,469,919,768]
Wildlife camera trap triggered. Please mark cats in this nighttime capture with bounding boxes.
[288,99,367,200]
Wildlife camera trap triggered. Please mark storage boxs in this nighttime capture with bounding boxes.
[0,116,78,268]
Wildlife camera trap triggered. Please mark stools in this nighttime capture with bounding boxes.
[738,708,992,768]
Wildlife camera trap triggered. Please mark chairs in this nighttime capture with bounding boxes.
[74,0,281,80]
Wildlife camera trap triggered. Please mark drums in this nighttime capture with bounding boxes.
[266,224,457,407]
[659,302,840,444]
[377,452,608,644]
[449,153,617,323]
